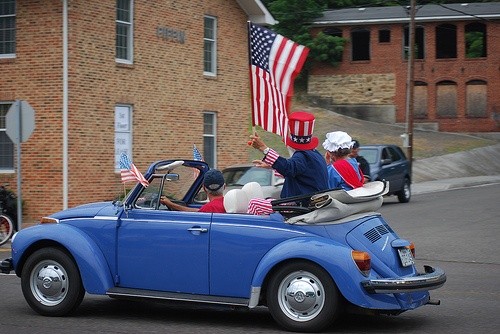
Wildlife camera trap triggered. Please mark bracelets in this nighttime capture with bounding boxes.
[264,148,269,154]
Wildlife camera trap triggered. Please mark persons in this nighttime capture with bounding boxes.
[160,169,226,213]
[348,140,371,183]
[322,132,365,190]
[247,112,329,215]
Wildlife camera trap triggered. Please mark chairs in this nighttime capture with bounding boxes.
[223,183,282,213]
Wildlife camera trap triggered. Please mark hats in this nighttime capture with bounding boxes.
[203,169,224,191]
[322,131,354,153]
[282,111,319,150]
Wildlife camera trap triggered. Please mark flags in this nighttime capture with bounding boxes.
[249,22,310,148]
[120,153,150,187]
[193,146,204,181]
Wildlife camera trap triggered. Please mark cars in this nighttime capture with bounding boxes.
[357,144,411,203]
[0,159,446,332]
[196,164,285,202]
